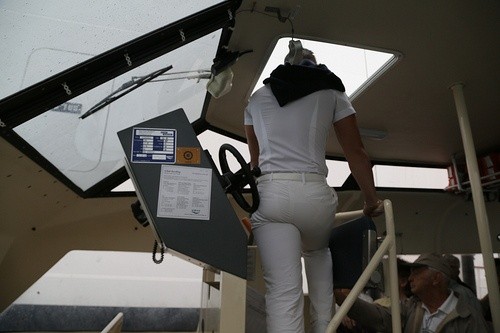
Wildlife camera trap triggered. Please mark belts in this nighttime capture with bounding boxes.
[257,173,325,182]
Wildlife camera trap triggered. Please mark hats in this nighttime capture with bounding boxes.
[409,253,450,277]
[443,254,460,273]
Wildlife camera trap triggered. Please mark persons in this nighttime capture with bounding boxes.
[243,40,383,333]
[338,251,491,333]
